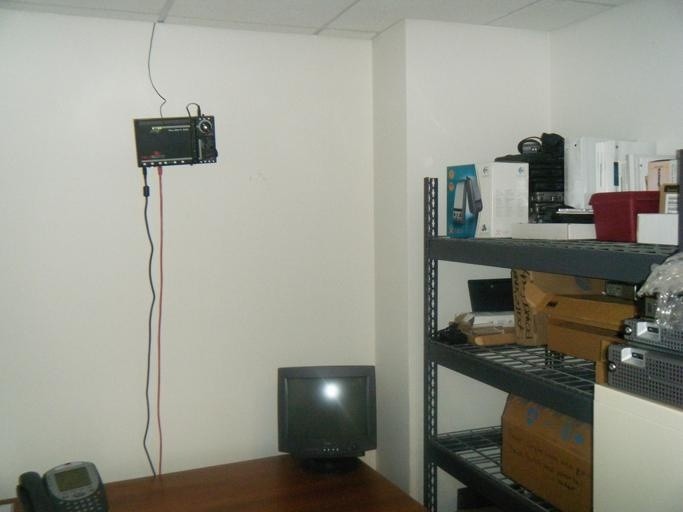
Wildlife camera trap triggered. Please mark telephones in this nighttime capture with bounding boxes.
[16,462,106,512]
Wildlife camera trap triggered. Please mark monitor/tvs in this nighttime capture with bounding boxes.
[277,366,377,459]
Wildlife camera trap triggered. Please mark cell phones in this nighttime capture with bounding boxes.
[452,180,467,224]
[466,175,482,214]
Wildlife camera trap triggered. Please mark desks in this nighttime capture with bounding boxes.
[0,455,423,511]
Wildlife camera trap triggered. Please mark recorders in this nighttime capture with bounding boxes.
[134,116,218,167]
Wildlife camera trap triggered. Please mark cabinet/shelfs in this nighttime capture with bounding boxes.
[422,175,683,512]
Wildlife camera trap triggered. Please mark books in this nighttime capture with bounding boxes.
[556,136,679,214]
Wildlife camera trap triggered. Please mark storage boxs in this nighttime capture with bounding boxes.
[590,189,659,242]
[498,393,594,511]
[510,268,647,381]
